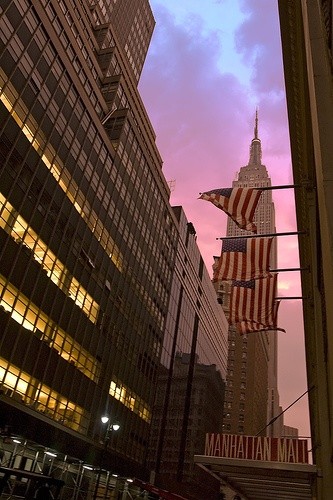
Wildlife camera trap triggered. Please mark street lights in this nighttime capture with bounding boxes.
[91,407,121,500]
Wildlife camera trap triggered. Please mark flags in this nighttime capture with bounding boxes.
[210,238,273,284]
[197,187,262,234]
[227,272,278,327]
[239,299,287,336]
